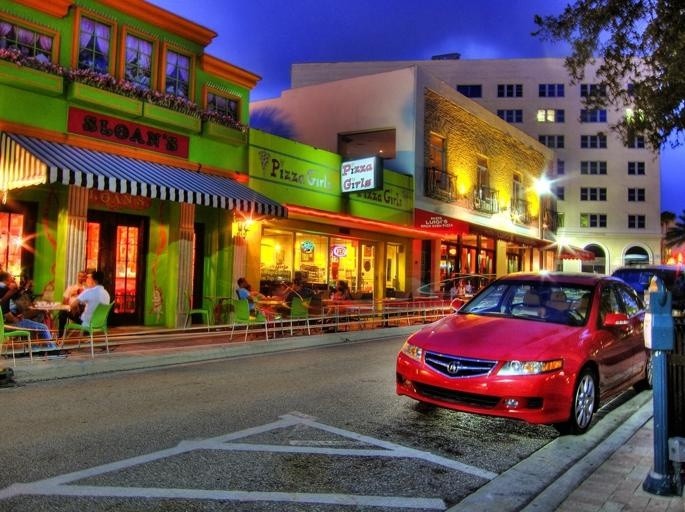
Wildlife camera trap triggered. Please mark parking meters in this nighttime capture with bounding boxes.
[643,276,675,495]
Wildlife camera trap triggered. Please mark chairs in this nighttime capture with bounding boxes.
[513,291,570,319]
[0,300,116,367]
[183,291,312,343]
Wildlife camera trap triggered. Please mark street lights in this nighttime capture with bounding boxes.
[538,181,546,270]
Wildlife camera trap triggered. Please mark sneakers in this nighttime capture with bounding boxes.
[48,349,68,356]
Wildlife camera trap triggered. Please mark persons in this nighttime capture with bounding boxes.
[318,286,350,321]
[0,269,65,356]
[567,291,604,324]
[61,270,88,307]
[448,277,475,298]
[236,276,261,316]
[244,282,267,301]
[277,277,321,315]
[322,281,353,332]
[58,270,111,340]
